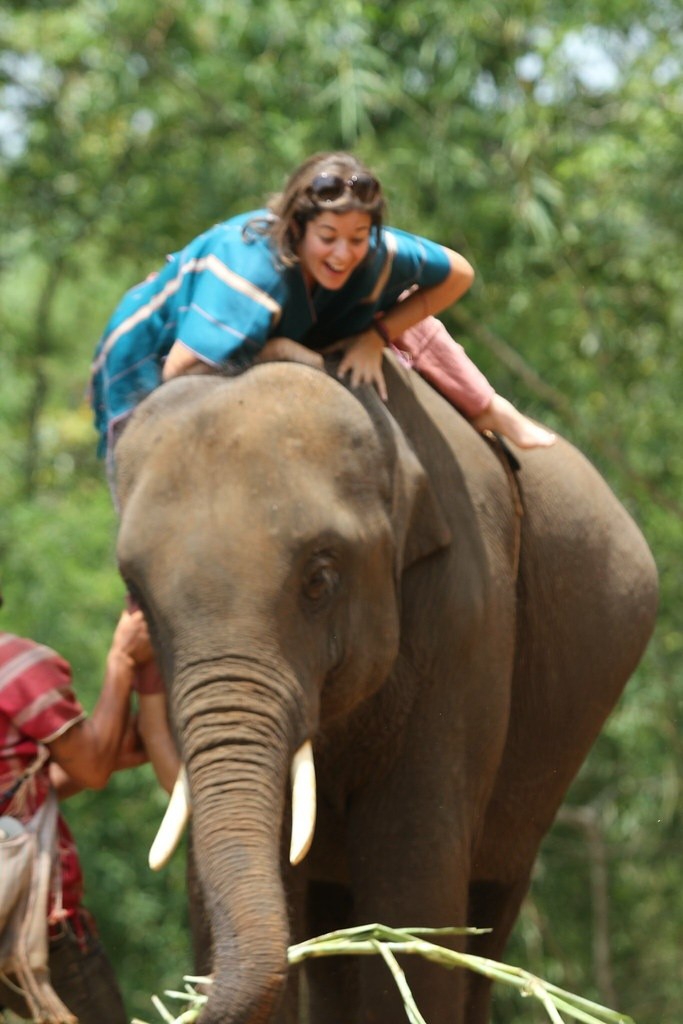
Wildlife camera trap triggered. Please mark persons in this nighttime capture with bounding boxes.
[0,597,156,1024]
[90,151,558,796]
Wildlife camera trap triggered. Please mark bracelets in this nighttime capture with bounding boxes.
[374,319,392,346]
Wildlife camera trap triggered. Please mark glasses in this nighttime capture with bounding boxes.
[310,172,381,204]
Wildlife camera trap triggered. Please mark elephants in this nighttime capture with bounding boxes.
[112,353,660,1024]
